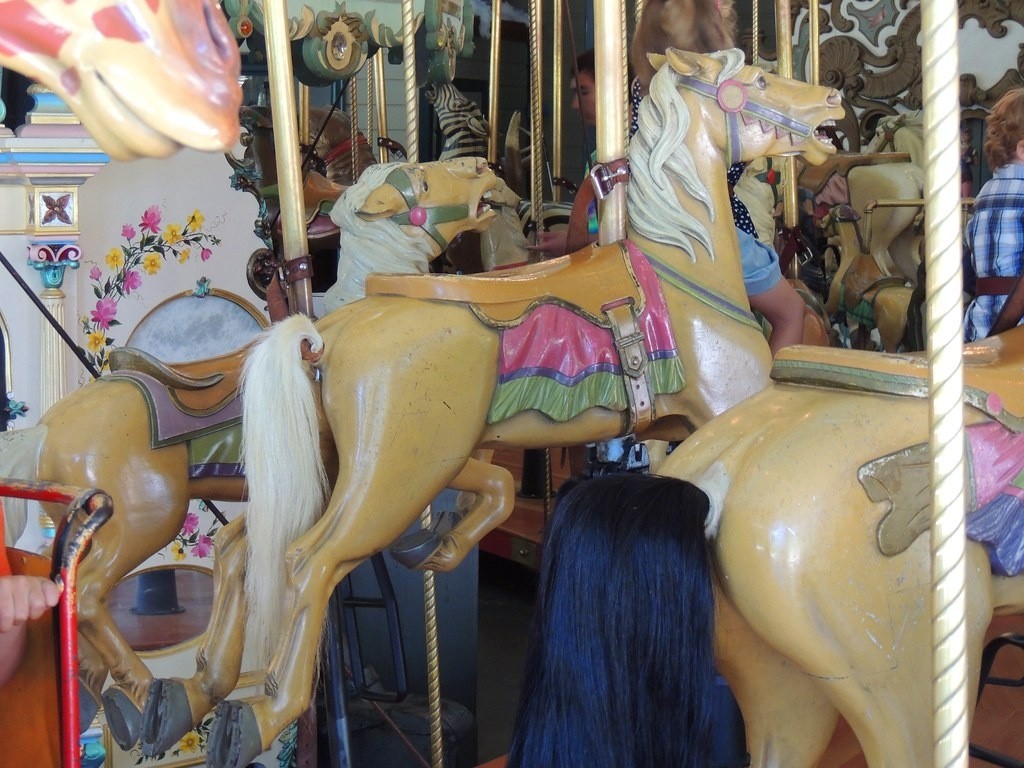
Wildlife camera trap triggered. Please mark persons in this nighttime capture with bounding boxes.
[1,499,62,684]
[505,471,751,767]
[626,0,809,365]
[524,49,598,258]
[962,85,1024,344]
[961,120,980,199]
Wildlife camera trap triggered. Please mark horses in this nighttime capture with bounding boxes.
[0,0,1024,768]
